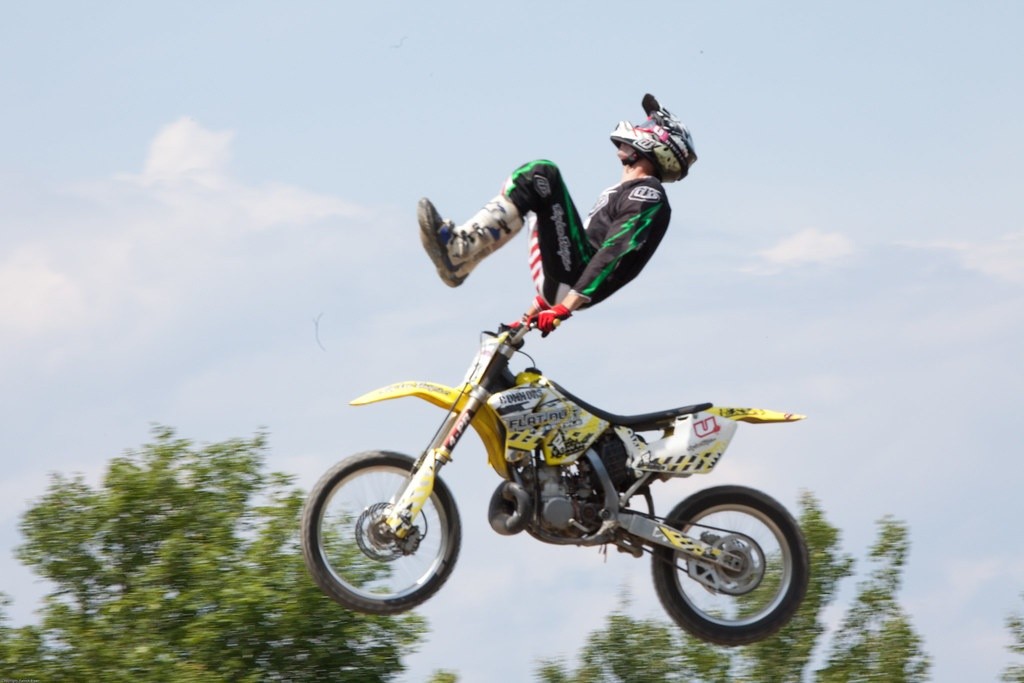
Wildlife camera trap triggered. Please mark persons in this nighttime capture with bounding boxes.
[418,93,697,340]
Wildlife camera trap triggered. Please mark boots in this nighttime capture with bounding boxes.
[416,193,525,288]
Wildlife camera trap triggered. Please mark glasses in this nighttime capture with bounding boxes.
[633,113,670,143]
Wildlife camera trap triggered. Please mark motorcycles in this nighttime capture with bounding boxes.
[301,319,811,647]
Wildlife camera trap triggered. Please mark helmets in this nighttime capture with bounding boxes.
[610,93,699,183]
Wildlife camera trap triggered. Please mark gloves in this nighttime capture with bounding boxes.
[525,302,571,334]
